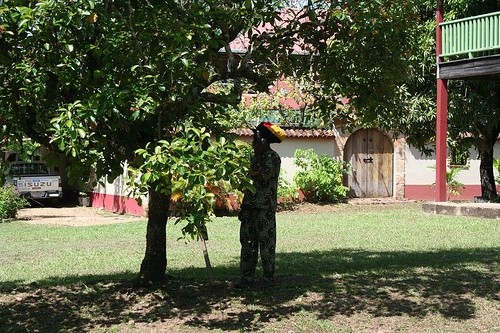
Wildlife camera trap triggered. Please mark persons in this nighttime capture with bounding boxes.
[230,121,286,290]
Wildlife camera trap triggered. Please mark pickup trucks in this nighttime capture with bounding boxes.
[4,160,63,207]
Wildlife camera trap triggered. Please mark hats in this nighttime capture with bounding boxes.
[251,122,286,143]
